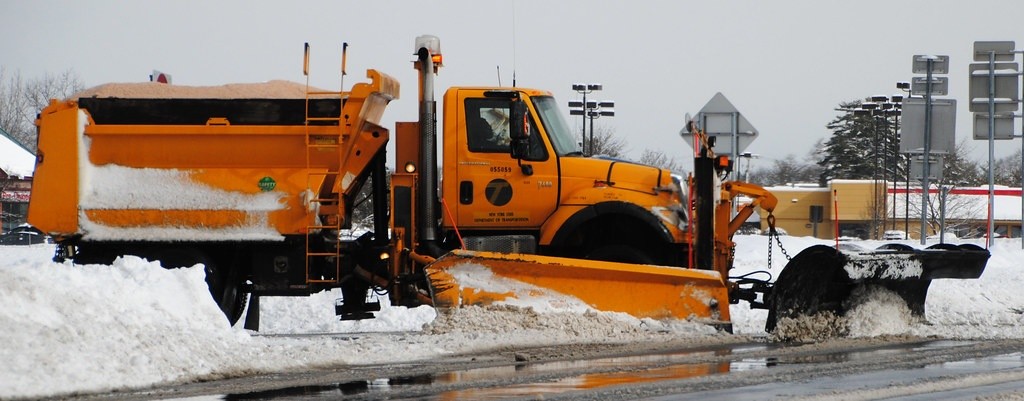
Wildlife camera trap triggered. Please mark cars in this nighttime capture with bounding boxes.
[0,226,45,244]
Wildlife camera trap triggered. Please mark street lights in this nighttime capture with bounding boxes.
[572,82,603,153]
[567,100,615,155]
[853,80,914,238]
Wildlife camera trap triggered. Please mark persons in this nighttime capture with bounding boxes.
[478,117,498,149]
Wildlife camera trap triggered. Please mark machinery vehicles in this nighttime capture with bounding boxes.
[25,34,992,333]
